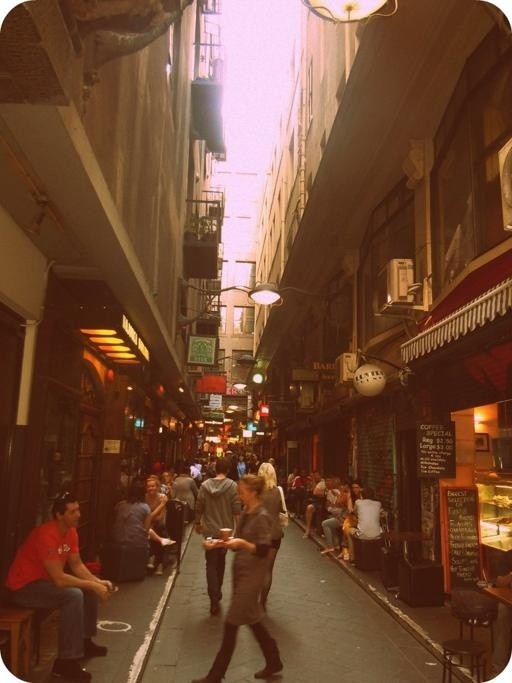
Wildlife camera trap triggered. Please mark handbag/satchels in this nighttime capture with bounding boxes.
[278,486,288,527]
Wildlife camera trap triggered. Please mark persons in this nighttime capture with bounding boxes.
[190,472,284,683]
[256,462,284,614]
[194,456,242,615]
[113,446,386,576]
[3,488,121,683]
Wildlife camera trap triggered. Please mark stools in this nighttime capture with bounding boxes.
[0,599,47,677]
[440,586,499,682]
[353,538,384,571]
[398,558,443,607]
[101,542,148,582]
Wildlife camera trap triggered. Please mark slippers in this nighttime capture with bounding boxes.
[302,533,309,539]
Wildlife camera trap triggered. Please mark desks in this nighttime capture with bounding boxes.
[381,531,433,599]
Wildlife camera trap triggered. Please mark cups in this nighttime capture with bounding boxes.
[219,527,233,542]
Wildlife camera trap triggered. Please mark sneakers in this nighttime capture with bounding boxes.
[50,659,91,682]
[161,538,176,546]
[154,563,163,575]
[83,638,107,658]
[321,545,354,562]
[147,554,156,569]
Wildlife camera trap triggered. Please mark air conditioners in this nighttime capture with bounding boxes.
[376,257,415,313]
[334,351,356,385]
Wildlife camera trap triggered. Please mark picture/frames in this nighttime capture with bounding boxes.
[474,432,489,452]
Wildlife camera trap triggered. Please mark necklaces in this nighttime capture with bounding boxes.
[491,547,511,589]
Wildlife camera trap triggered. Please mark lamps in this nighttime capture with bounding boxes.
[247,279,336,306]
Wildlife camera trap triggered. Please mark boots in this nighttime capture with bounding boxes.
[191,653,231,683]
[255,639,282,678]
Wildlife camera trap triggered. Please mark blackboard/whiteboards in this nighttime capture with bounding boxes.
[437,485,483,580]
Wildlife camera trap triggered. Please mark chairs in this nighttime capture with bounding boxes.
[166,499,185,575]
